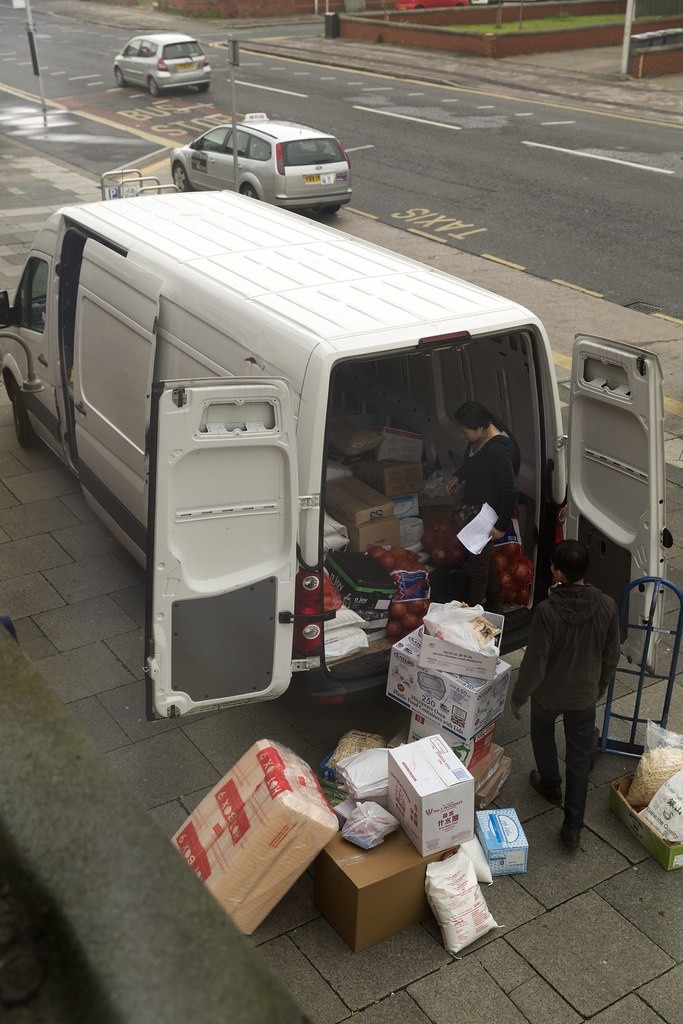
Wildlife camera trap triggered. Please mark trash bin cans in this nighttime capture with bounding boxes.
[325,11,340,39]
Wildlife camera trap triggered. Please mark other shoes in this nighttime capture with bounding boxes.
[560,826,580,847]
[530,768,563,804]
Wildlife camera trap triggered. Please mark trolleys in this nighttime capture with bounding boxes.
[598,576,683,758]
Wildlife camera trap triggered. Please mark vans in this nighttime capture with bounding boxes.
[0,188,667,724]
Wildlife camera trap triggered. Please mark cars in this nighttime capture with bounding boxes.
[169,113,353,215]
[112,31,212,97]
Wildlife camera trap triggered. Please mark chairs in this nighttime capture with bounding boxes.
[291,142,302,154]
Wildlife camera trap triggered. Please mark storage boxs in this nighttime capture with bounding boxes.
[418,602,504,680]
[327,510,400,553]
[386,624,513,743]
[419,497,457,529]
[400,516,424,546]
[325,551,397,611]
[353,460,424,497]
[326,459,352,482]
[326,476,393,526]
[391,492,420,518]
[374,426,423,464]
[163,713,683,954]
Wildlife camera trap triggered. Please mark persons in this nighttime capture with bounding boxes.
[511,540,621,849]
[445,402,517,613]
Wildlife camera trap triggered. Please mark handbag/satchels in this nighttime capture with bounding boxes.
[451,500,480,529]
